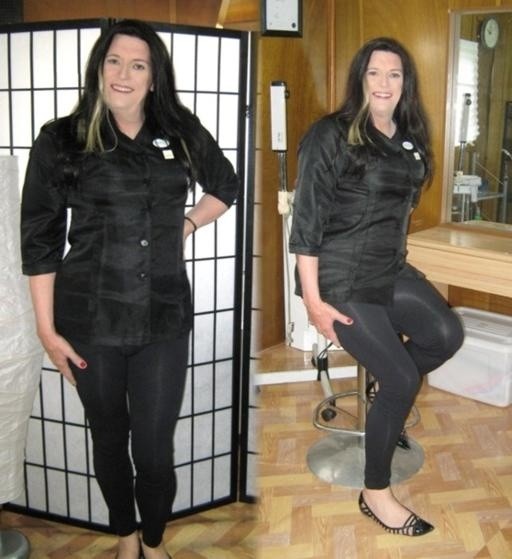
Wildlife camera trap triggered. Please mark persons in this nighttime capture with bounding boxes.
[287,36,463,534]
[21,17,241,559]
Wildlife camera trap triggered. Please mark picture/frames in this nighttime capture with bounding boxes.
[259,0,303,39]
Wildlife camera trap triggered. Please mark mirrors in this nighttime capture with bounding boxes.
[442,7,512,241]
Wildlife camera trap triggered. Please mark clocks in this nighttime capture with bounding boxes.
[477,16,501,50]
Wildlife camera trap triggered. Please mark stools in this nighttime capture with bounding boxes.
[308,324,424,489]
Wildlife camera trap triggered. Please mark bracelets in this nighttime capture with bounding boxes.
[183,216,198,231]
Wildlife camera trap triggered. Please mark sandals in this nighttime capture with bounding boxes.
[364,380,411,452]
[358,488,435,537]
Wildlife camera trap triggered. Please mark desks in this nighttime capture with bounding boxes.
[405,226,512,306]
